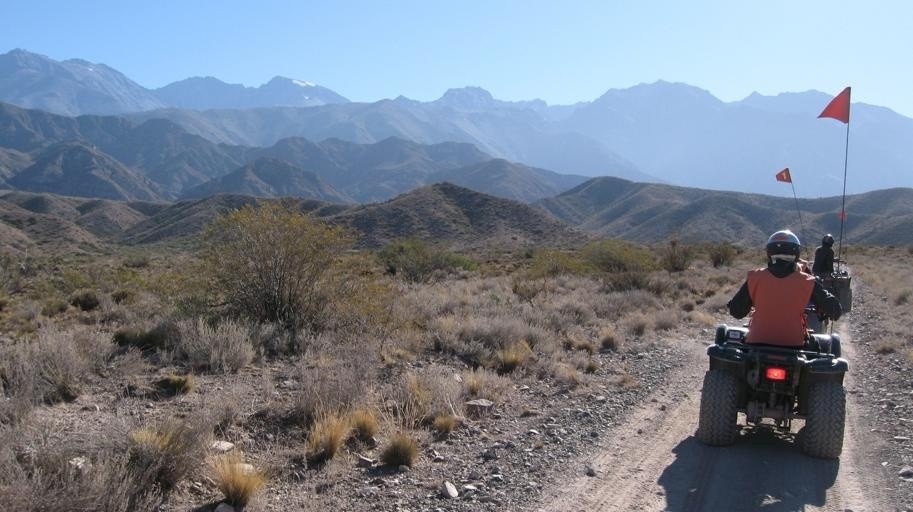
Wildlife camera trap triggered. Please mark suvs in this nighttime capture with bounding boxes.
[696,301,850,460]
[830,267,853,312]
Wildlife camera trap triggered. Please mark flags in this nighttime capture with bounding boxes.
[776,169,791,183]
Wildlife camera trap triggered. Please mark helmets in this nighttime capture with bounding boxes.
[766,231,801,264]
[822,234,834,246]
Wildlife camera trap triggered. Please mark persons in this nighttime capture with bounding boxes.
[812,234,834,273]
[729,231,842,347]
